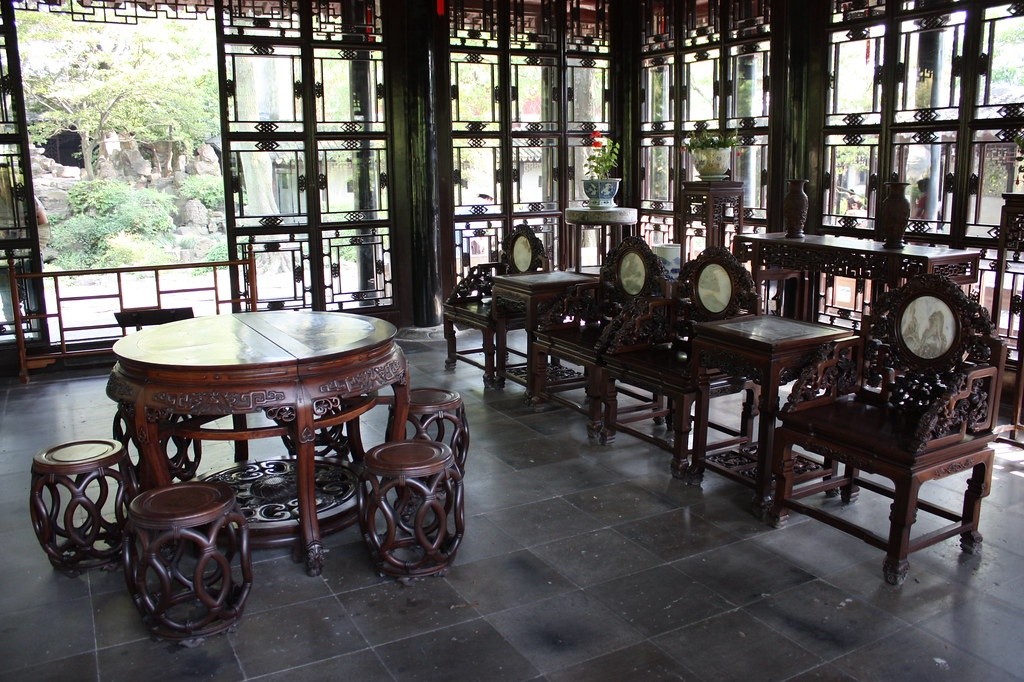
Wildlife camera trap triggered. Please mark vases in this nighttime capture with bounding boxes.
[783,178,809,238]
[653,243,681,281]
[581,178,622,209]
[880,181,912,249]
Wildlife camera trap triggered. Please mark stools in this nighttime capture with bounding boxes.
[29,439,138,580]
[275,406,349,457]
[385,388,470,501]
[113,410,203,493]
[121,482,254,642]
[356,440,465,580]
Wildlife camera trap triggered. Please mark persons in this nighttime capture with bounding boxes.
[846,189,856,209]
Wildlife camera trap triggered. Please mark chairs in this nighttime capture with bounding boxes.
[529,236,673,438]
[443,224,549,389]
[765,273,1008,587]
[596,246,762,479]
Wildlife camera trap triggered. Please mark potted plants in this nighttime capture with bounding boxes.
[683,127,743,181]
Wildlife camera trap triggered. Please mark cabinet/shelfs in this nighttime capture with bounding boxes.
[565,208,638,273]
[680,181,745,266]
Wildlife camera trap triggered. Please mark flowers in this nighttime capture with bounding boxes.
[584,131,620,179]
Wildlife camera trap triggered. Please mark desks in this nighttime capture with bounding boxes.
[685,316,853,519]
[732,231,980,336]
[105,310,410,577]
[491,270,599,406]
